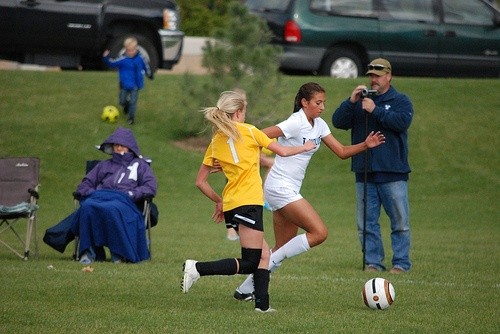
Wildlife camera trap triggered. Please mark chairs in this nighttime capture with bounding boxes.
[0,158,41,260]
[73,159,153,261]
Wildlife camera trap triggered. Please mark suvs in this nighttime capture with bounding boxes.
[0,0,185,71]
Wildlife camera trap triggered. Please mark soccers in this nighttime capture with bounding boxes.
[100,105,120,122]
[361,277,395,310]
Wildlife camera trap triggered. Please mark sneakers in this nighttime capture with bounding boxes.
[234,289,256,302]
[254,306,277,313]
[181,260,200,294]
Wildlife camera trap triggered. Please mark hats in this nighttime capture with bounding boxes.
[367,58,391,76]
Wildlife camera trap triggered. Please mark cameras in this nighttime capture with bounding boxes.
[359,89,378,100]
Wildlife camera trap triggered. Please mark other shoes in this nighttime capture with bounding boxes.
[366,268,377,272]
[80,255,91,264]
[390,268,403,273]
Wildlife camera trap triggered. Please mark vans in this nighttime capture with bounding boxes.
[280,0,499,80]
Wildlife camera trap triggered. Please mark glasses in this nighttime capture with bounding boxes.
[368,64,387,70]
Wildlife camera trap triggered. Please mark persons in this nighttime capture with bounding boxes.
[210,82,385,302]
[181,91,317,314]
[226,87,277,240]
[332,58,414,273]
[72,127,158,265]
[103,38,154,125]
